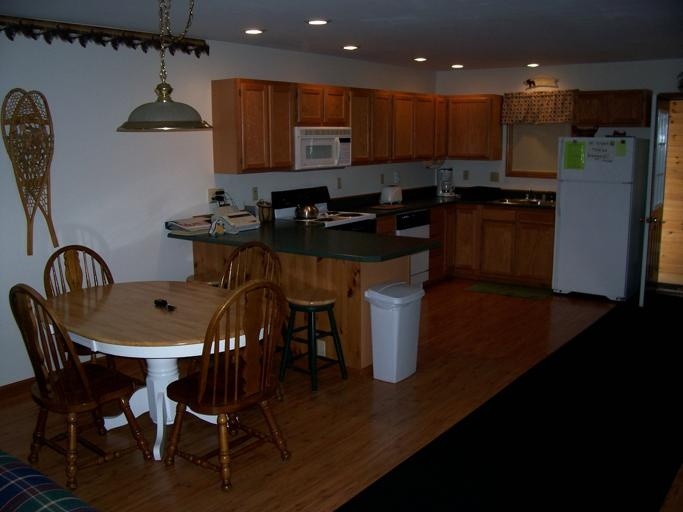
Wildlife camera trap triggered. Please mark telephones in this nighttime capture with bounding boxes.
[210,205,260,235]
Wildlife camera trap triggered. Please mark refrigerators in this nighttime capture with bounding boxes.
[551,137,650,300]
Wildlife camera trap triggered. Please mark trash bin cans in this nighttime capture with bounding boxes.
[363,280,425,384]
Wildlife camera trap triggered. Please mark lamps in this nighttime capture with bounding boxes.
[116,0,214,132]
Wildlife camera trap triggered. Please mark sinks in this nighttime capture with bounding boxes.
[496,198,556,206]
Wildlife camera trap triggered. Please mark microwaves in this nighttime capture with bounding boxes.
[295,127,352,170]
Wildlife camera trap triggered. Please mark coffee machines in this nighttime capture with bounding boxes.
[438,168,456,196]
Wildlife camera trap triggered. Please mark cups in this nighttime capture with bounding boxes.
[216,223,224,236]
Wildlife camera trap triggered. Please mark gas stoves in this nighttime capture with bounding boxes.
[280,210,376,223]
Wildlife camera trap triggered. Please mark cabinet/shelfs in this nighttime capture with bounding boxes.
[445,206,455,273]
[576,91,642,123]
[454,206,481,273]
[295,84,347,126]
[447,98,501,162]
[481,208,555,281]
[429,206,445,280]
[434,99,447,158]
[348,90,392,165]
[392,93,435,160]
[212,76,295,174]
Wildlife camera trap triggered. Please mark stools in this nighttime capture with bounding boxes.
[186,273,227,285]
[279,288,349,391]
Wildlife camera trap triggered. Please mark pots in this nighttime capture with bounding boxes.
[571,125,599,137]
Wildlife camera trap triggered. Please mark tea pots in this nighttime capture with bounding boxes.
[295,203,319,219]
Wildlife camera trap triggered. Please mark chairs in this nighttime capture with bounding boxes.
[9,283,153,490]
[163,279,291,492]
[187,241,283,402]
[44,244,148,391]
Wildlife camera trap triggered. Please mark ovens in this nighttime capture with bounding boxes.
[396,209,429,284]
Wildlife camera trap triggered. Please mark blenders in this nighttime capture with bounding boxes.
[380,171,402,205]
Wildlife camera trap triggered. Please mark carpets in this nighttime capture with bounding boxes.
[463,280,553,301]
[332,288,683,511]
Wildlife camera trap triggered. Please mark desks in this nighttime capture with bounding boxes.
[38,280,272,460]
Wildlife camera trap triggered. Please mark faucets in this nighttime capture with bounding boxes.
[524,182,538,201]
